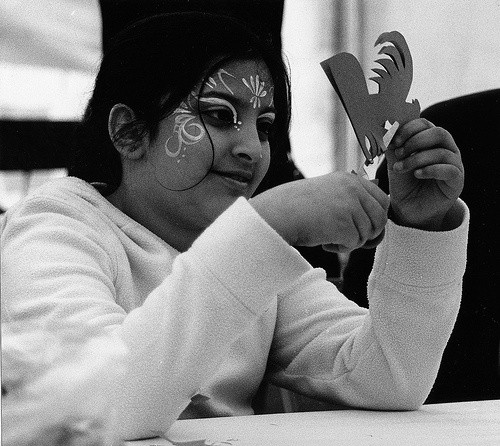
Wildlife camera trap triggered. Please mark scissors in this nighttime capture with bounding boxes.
[322,118,400,252]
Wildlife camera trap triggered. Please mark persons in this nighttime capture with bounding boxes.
[0,0,470,446]
